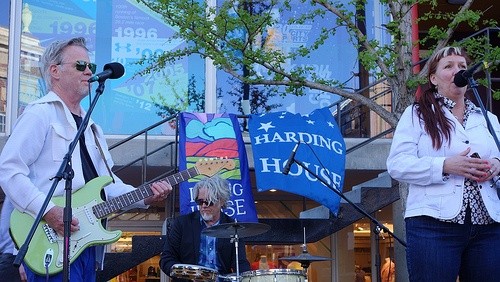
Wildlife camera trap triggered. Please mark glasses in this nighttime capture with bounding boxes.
[55,60,97,75]
[194,197,213,207]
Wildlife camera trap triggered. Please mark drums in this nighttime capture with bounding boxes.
[241,269,307,282]
[170,264,216,282]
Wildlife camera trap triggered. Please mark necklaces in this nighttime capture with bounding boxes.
[455,116,463,120]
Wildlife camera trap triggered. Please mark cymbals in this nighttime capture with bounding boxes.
[201,222,271,238]
[278,253,335,266]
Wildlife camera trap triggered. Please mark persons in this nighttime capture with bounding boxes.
[385,47,500,282]
[0,37,173,282]
[158,175,251,282]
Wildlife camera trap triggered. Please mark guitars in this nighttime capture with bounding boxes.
[8,156,236,278]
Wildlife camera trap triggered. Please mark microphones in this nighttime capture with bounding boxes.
[87,62,125,82]
[454,61,483,87]
[282,140,300,175]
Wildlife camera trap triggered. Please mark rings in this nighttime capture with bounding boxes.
[486,169,493,175]
[161,193,165,196]
